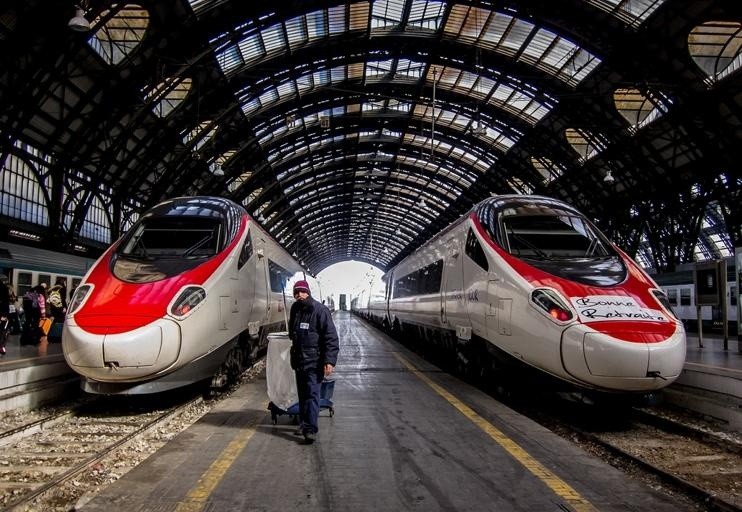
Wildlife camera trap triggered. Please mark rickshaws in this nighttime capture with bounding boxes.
[61,194,324,397]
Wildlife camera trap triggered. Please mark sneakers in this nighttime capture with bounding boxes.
[0,345,6,355]
[292,423,317,442]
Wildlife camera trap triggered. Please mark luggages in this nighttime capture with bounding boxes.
[0,316,9,350]
[19,318,43,347]
[47,321,64,343]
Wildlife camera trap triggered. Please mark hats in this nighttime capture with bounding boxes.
[292,279,310,297]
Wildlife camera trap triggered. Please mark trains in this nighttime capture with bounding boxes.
[352,194,686,406]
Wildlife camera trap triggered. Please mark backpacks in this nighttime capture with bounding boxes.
[49,288,63,308]
[21,289,39,312]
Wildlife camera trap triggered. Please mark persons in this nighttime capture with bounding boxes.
[288,280,340,442]
[0,274,68,347]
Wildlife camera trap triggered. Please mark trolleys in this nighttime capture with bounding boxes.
[266,329,335,424]
